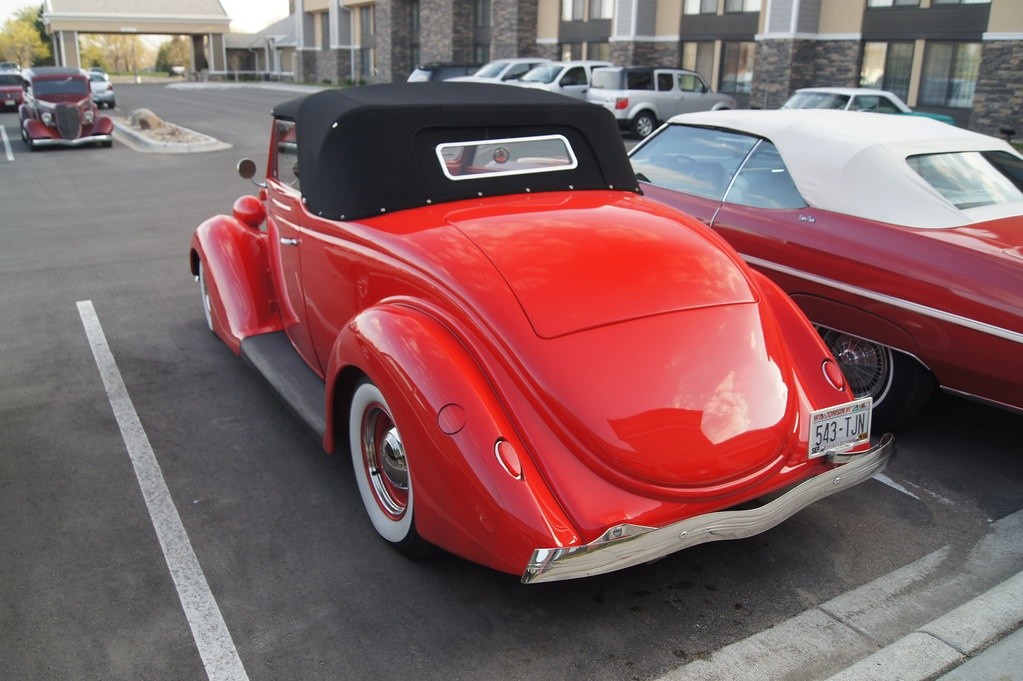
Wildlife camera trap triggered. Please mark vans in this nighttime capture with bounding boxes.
[579,65,735,140]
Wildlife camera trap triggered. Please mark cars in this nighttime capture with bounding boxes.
[87,72,117,108]
[496,60,616,101]
[184,79,897,585]
[406,59,484,83]
[442,57,554,85]
[0,61,28,115]
[17,65,115,148]
[768,83,921,120]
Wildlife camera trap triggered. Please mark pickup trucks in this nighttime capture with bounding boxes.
[475,102,1022,444]
